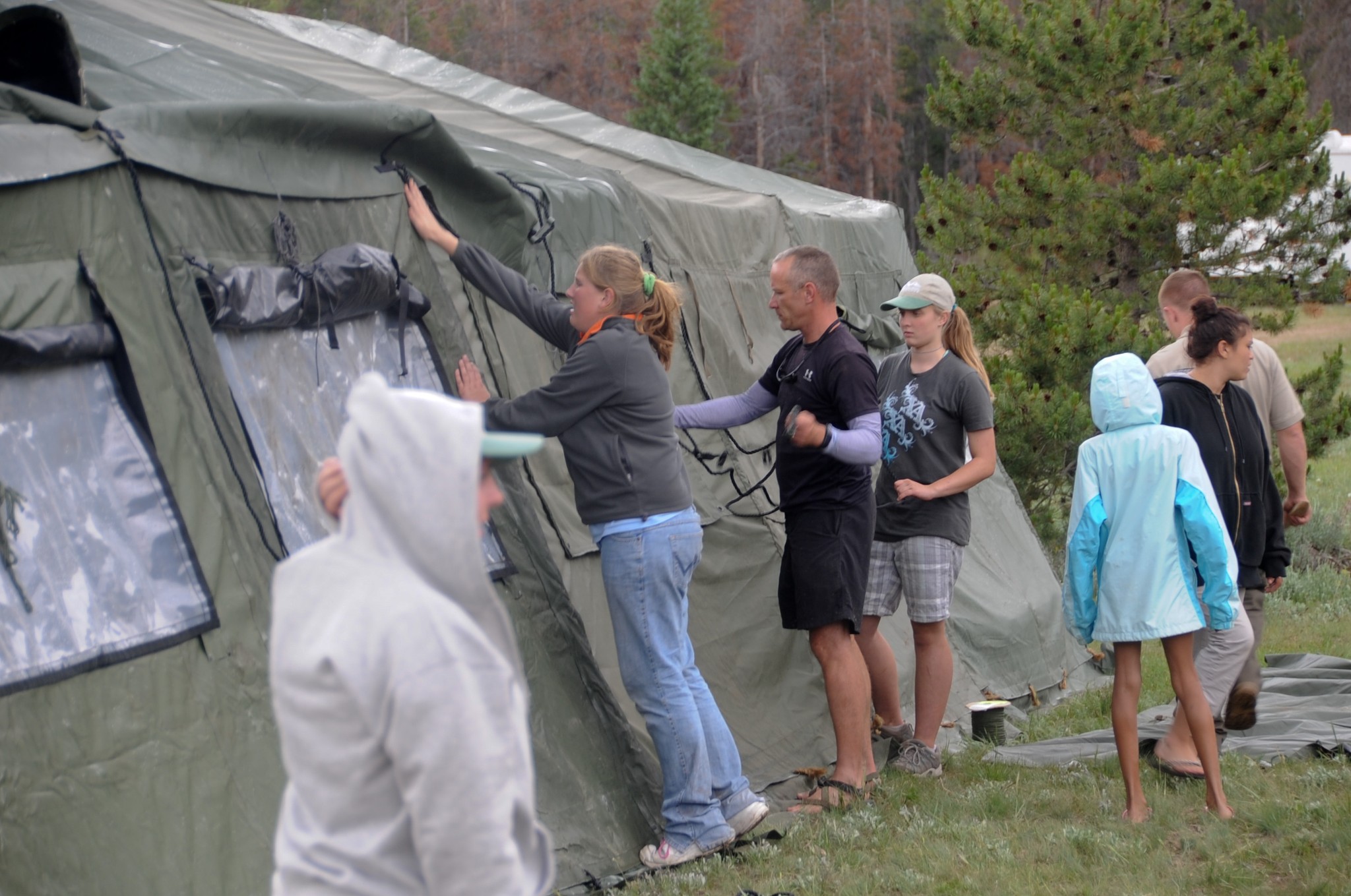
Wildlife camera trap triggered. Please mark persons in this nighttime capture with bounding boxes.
[674,248,882,817]
[406,177,768,870]
[854,273,996,780]
[1063,351,1239,827]
[271,372,558,893]
[1142,269,1312,755]
[1147,293,1290,780]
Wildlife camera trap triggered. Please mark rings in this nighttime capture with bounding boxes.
[792,432,795,439]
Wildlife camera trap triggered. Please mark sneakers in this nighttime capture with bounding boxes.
[871,723,914,766]
[724,801,770,838]
[888,739,943,777]
[639,827,736,867]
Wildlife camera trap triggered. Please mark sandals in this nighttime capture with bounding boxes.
[794,770,884,800]
[786,775,871,819]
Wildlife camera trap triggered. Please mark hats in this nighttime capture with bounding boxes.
[480,430,546,459]
[879,273,956,312]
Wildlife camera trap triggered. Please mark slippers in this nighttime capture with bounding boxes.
[1152,758,1205,780]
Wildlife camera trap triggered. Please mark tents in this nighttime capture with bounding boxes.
[0,0,1113,896]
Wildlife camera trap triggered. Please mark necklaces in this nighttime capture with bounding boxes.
[910,343,944,353]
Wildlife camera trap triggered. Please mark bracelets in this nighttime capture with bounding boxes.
[817,424,832,450]
